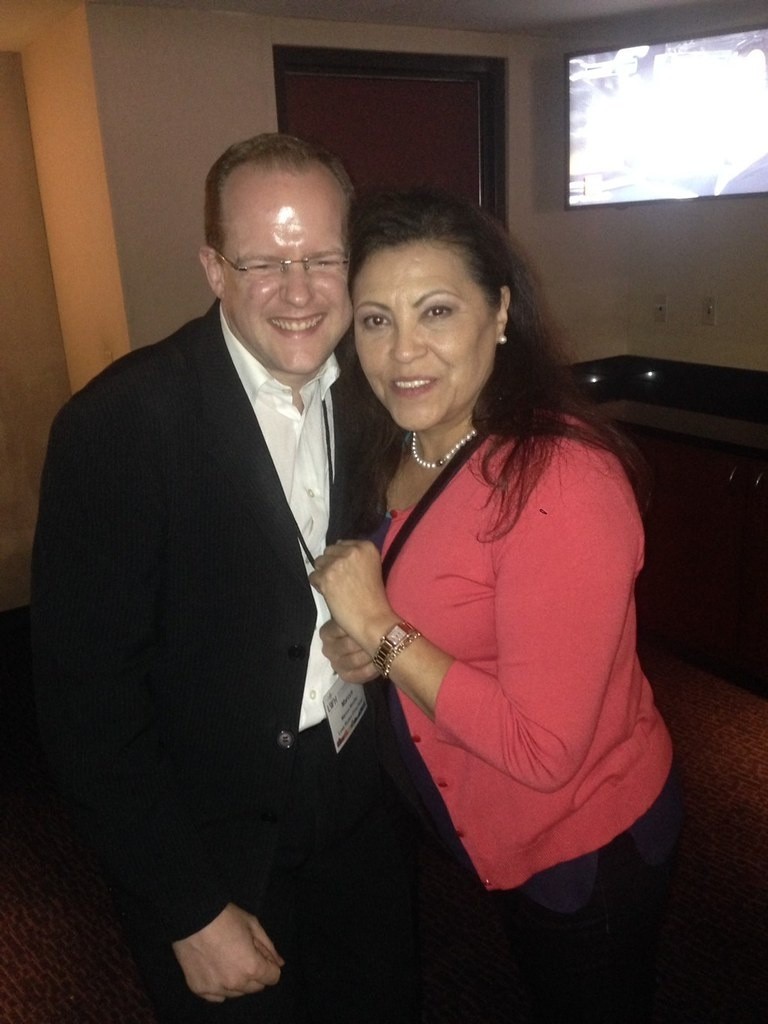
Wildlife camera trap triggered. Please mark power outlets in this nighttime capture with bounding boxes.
[653,295,668,323]
[703,295,716,326]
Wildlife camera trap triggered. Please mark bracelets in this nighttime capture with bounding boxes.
[383,632,420,679]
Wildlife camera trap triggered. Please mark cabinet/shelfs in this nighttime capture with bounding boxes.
[562,355,768,702]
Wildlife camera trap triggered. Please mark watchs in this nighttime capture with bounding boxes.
[374,622,414,670]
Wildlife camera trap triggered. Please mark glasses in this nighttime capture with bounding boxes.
[216,242,350,285]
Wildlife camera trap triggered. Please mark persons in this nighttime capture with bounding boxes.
[32,135,424,1024]
[310,189,681,1024]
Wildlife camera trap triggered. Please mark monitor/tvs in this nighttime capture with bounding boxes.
[563,23,768,209]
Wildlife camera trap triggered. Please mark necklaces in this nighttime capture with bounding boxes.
[411,430,480,469]
